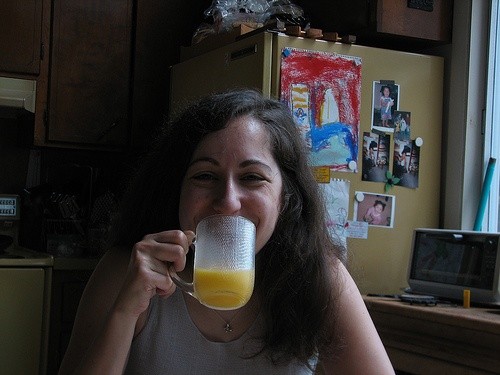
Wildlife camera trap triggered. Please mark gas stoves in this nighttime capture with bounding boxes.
[0,194,54,266]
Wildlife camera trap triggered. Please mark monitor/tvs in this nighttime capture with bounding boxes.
[406,226,500,304]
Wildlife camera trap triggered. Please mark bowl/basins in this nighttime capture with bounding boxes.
[0,234,15,252]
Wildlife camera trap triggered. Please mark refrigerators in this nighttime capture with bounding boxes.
[166,30,445,297]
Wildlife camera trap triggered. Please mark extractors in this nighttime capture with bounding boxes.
[0,76,37,113]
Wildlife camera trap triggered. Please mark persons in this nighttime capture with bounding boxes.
[400,117,406,138]
[364,200,386,225]
[57,89,396,375]
[400,152,406,176]
[378,86,394,127]
[369,148,375,167]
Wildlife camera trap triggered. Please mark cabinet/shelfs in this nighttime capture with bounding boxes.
[313,294,500,375]
[0,0,216,153]
[304,0,454,55]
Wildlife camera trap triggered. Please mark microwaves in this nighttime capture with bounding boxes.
[407,228,500,304]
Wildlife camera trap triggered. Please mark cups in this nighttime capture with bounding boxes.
[165,214,255,310]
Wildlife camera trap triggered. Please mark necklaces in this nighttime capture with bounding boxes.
[215,299,252,332]
